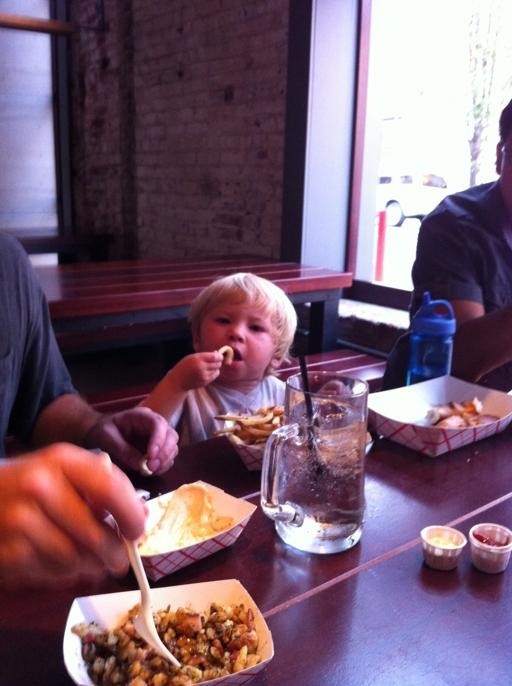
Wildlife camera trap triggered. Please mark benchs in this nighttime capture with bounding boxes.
[87,346,388,407]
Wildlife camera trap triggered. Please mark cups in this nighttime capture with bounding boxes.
[420,523,512,576]
[259,370,370,555]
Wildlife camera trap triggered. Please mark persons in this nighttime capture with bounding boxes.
[135,271,298,448]
[1,227,180,597]
[382,97,510,391]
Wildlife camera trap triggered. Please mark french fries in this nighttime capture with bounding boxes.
[214,407,285,444]
[218,345,233,363]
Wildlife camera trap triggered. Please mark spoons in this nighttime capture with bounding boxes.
[90,448,182,674]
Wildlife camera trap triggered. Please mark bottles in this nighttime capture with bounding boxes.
[406,293,455,384]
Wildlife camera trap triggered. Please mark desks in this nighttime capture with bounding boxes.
[28,254,354,353]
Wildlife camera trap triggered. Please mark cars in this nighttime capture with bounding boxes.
[378,174,447,226]
